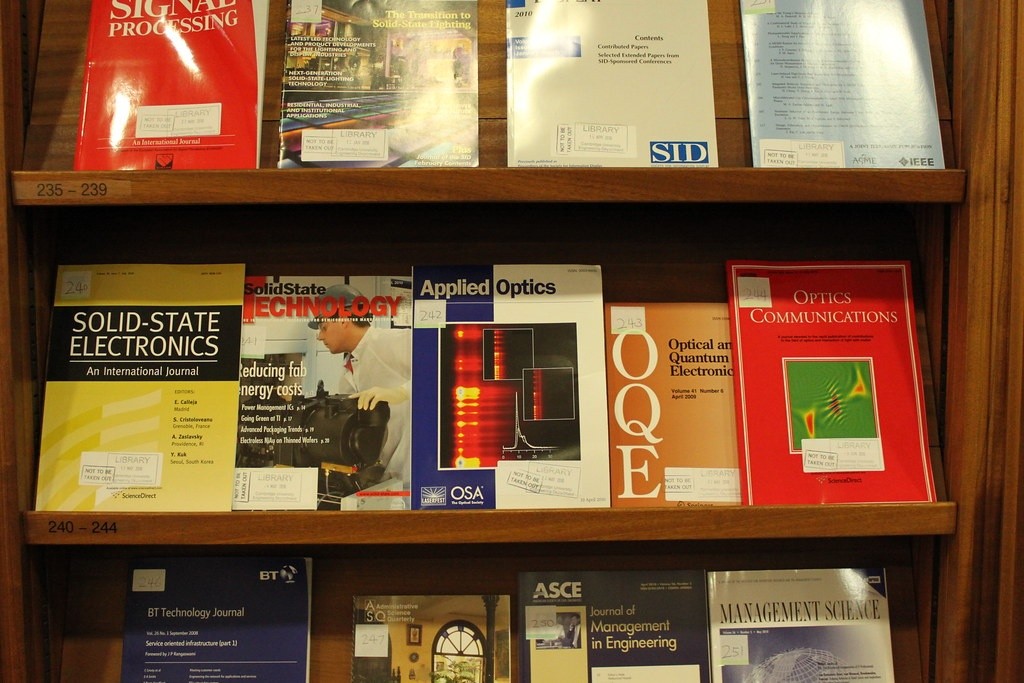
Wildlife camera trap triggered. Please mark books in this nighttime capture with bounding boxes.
[75,1,266,170]
[518,570,711,683]
[349,592,511,683]
[277,1,480,167]
[505,0,719,167]
[120,554,311,683]
[738,1,945,170]
[34,262,940,505]
[708,568,897,683]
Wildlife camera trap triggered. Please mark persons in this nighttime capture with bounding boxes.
[302,283,413,483]
[567,614,581,648]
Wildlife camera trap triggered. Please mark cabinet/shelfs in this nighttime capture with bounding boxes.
[0,2,1024,683]
